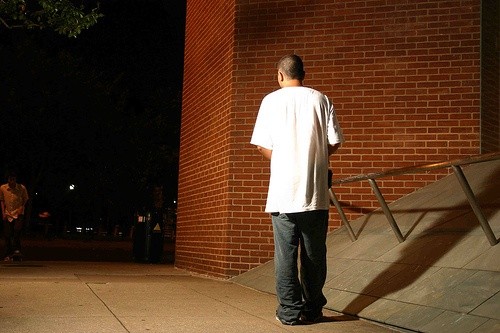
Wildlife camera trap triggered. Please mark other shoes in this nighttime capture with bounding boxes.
[275,310,323,325]
[13,249,21,253]
[3,256,10,264]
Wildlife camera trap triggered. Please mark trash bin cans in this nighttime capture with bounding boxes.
[150,207,176,263]
[130,205,156,263]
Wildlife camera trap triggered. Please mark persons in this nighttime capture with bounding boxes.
[0,175,30,264]
[249,53,342,325]
